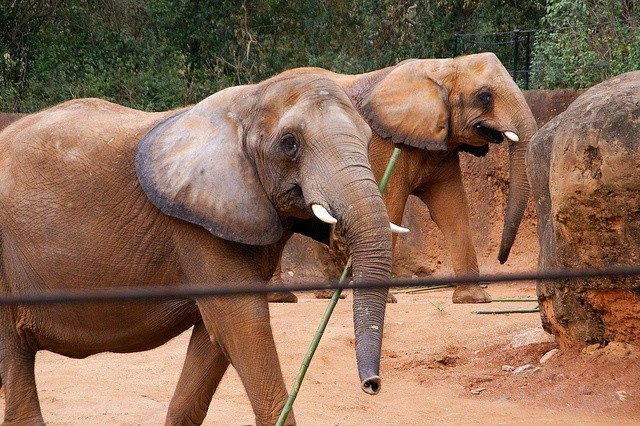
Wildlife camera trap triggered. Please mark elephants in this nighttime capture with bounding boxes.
[0,73,409,426]
[267,52,538,303]
